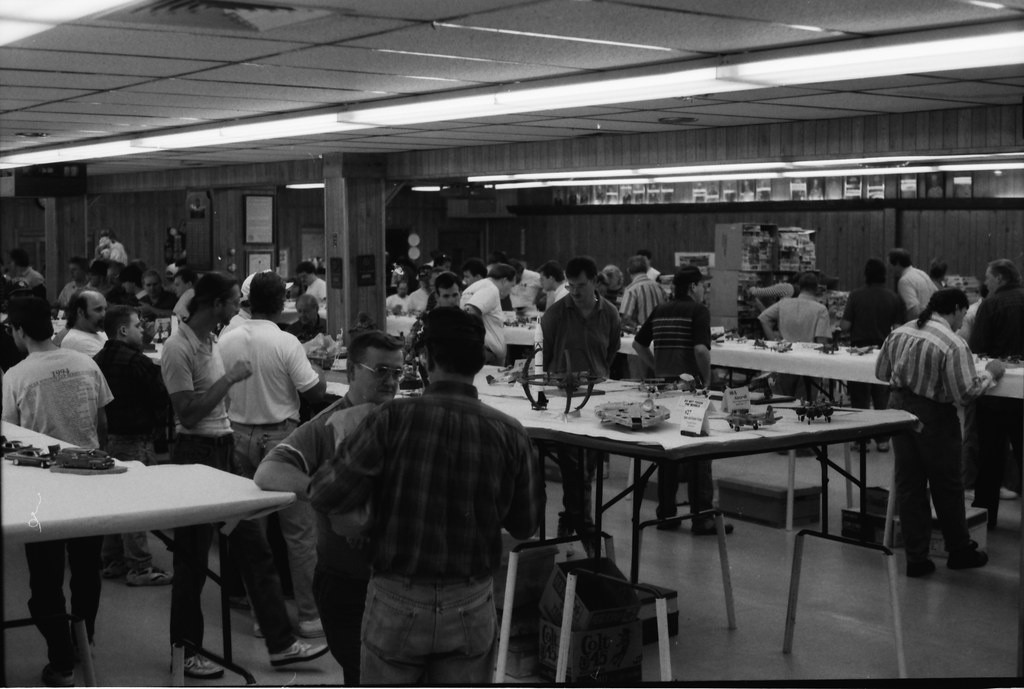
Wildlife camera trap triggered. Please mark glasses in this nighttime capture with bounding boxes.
[6,322,19,337]
[349,359,407,386]
[565,276,592,291]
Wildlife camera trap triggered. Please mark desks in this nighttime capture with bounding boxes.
[1,304,1024,688]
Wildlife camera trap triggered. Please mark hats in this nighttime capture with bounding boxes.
[3,297,52,322]
[414,309,485,345]
[674,266,713,283]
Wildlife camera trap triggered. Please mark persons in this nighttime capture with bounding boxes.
[254,330,406,686]
[304,305,538,685]
[840,250,985,491]
[0,296,114,688]
[385,248,669,369]
[0,227,330,676]
[537,256,621,558]
[749,269,834,403]
[876,288,1004,578]
[633,265,733,535]
[970,259,1024,530]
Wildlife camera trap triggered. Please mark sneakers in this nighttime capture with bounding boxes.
[270,639,328,666]
[170,651,225,679]
[104,562,129,579]
[126,565,173,588]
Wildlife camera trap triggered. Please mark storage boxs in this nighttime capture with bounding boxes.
[840,506,903,549]
[640,579,679,646]
[486,533,559,606]
[539,615,640,684]
[495,634,536,681]
[719,476,823,528]
[539,557,640,630]
[927,508,987,561]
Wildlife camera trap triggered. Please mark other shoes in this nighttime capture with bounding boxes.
[42,663,76,687]
[946,541,988,569]
[878,442,890,451]
[850,441,870,452]
[253,619,264,639]
[659,515,683,530]
[691,519,732,536]
[999,486,1019,499]
[907,561,935,577]
[298,618,329,640]
